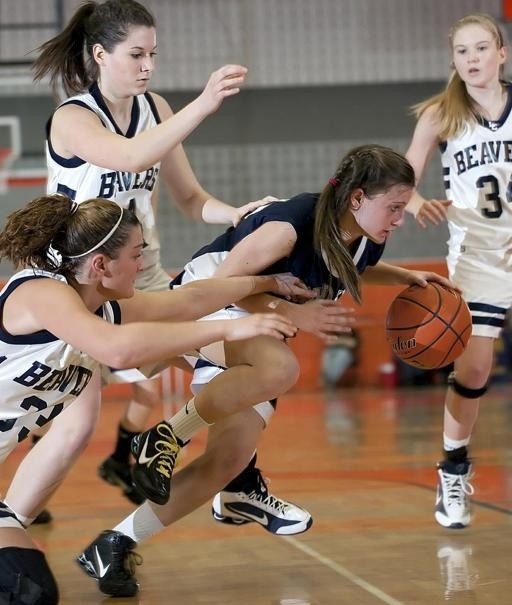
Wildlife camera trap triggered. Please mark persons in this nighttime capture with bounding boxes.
[73,141,463,597]
[31,0,314,538]
[0,190,318,604]
[403,12,512,528]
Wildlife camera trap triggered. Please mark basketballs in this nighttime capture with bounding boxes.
[388,283,472,369]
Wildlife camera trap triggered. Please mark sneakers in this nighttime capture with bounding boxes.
[433,460,475,529]
[211,468,314,536]
[131,420,191,506]
[98,455,146,506]
[75,529,143,597]
